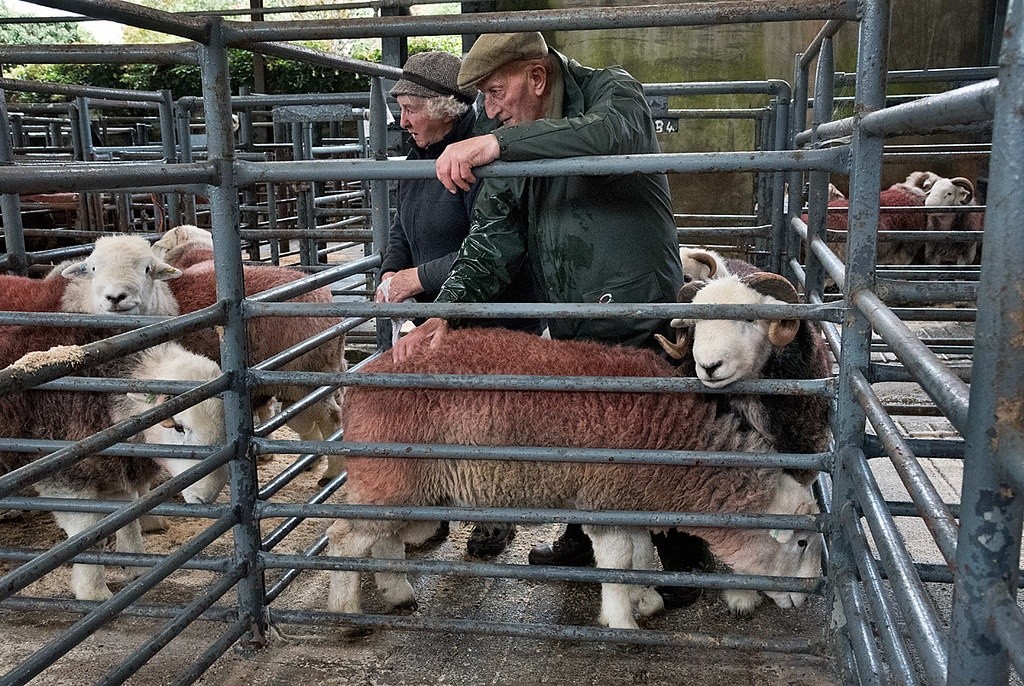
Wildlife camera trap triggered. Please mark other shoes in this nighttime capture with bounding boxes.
[431,520,452,538]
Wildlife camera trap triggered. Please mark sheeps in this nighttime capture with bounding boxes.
[324,247,836,630]
[1,225,352,601]
[782,170,985,296]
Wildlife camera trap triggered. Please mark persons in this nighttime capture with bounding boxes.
[391,32,717,608]
[374,53,549,559]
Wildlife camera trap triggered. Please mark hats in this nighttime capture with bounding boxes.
[457,31,548,90]
[390,51,479,106]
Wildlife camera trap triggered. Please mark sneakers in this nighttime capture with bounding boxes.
[467,522,515,557]
[654,552,716,608]
[528,534,595,566]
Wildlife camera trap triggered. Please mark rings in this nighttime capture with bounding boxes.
[394,290,398,295]
